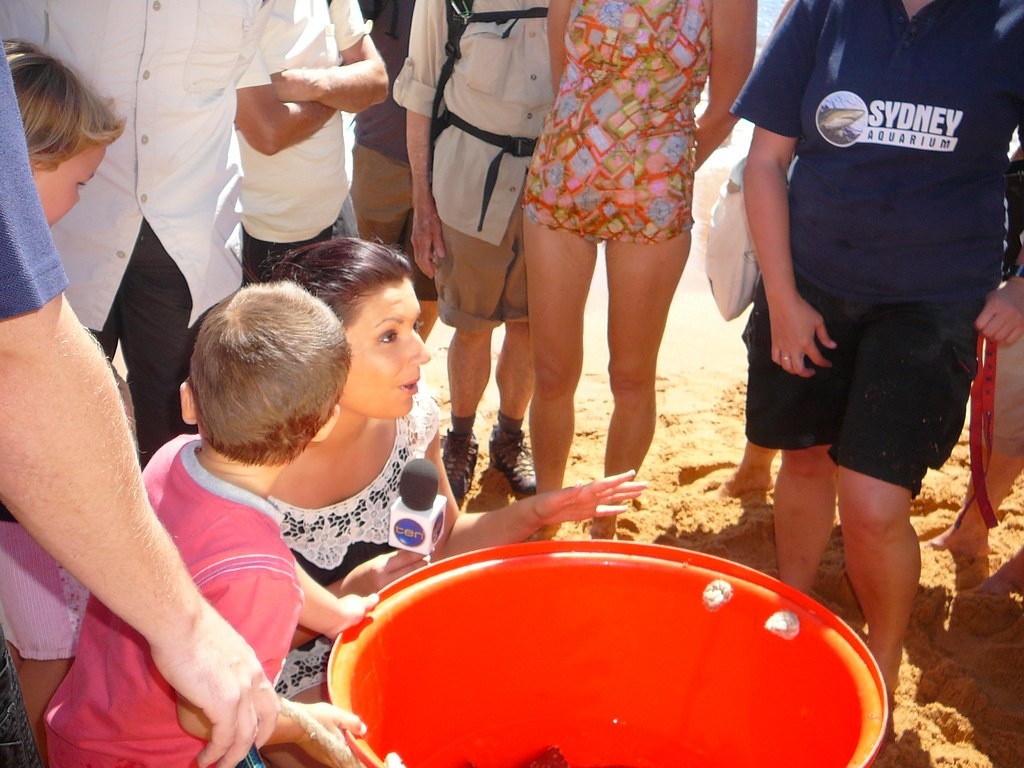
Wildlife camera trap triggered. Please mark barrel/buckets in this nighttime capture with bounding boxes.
[326,542,891,767]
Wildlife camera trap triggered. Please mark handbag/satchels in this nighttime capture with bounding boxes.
[701,148,764,324]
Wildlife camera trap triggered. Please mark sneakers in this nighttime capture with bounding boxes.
[489,426,539,495]
[438,430,477,498]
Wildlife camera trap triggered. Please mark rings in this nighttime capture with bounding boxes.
[781,357,789,361]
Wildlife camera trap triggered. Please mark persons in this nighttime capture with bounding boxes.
[44,284,379,768]
[0,0,277,471]
[0,41,123,768]
[719,0,1024,694]
[234,0,388,267]
[394,0,556,497]
[350,0,439,341]
[0,34,279,768]
[520,0,758,495]
[257,238,647,768]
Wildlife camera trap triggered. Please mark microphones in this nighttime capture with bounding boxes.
[388,459,447,554]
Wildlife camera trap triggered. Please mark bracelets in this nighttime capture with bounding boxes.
[1002,264,1024,280]
